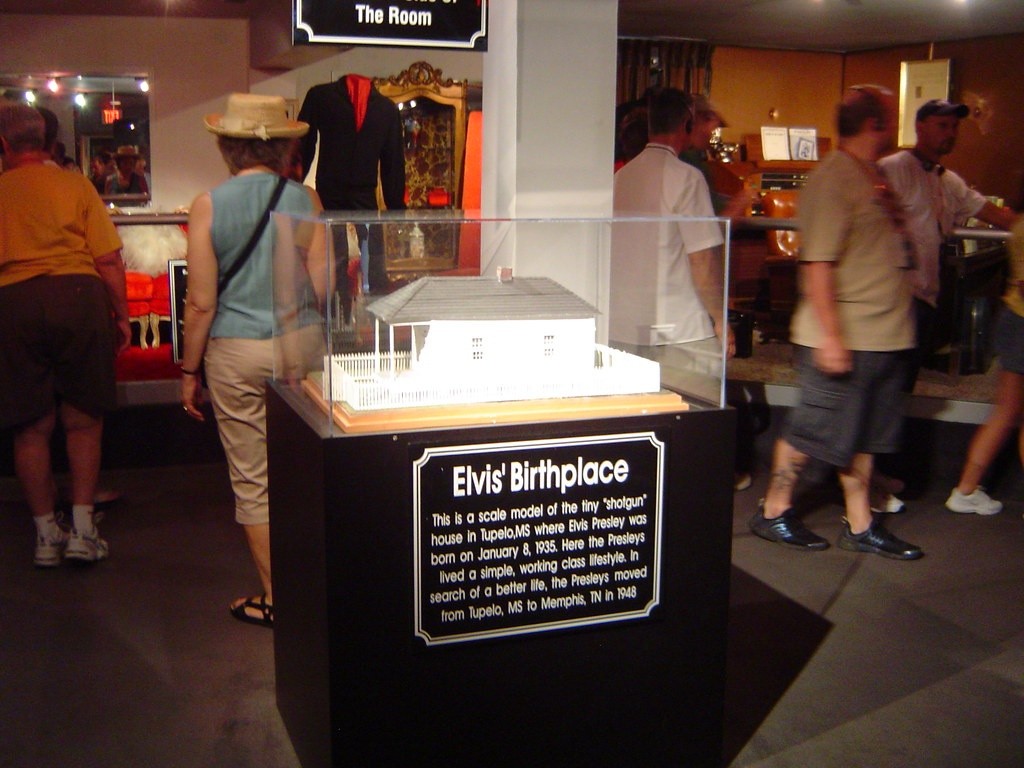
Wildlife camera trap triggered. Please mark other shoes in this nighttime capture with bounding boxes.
[735,471,751,490]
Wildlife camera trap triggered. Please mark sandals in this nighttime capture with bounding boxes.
[229,593,273,627]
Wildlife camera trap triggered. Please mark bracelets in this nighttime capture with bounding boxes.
[181,367,200,376]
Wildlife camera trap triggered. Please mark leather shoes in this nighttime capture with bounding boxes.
[748,508,830,550]
[837,520,921,559]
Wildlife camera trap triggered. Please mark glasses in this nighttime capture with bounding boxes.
[923,160,945,176]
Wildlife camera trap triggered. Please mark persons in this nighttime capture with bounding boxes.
[34,106,122,509]
[181,92,336,628]
[869,98,1017,512]
[610,85,789,492]
[750,84,923,560]
[0,96,132,568]
[293,74,408,323]
[53,141,151,195]
[944,211,1024,515]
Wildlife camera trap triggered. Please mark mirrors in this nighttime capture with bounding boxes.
[0,66,155,208]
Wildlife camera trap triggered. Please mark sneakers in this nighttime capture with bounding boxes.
[64,526,108,561]
[32,529,71,566]
[870,489,906,514]
[946,487,1003,515]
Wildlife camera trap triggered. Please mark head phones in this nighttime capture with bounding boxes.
[911,147,945,175]
[849,85,886,131]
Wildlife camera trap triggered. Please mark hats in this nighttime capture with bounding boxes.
[203,93,311,141]
[112,145,140,160]
[917,99,969,121]
